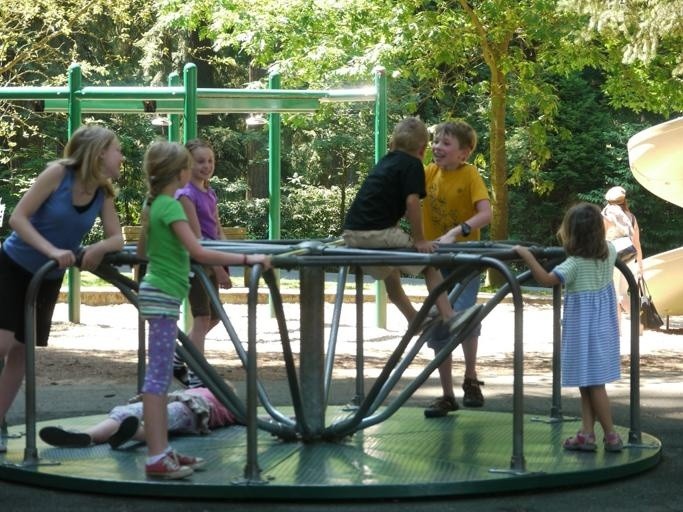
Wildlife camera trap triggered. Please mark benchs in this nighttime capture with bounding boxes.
[122,225,253,287]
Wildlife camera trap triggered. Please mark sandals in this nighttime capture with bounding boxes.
[562,429,597,451]
[422,395,459,419]
[170,363,206,390]
[601,428,623,453]
[460,375,485,407]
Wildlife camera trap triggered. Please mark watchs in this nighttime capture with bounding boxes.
[459,223,472,238]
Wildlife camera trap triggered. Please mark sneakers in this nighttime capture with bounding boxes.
[445,300,484,337]
[0,419,10,452]
[107,414,138,450]
[36,424,95,450]
[167,448,204,467]
[405,302,439,336]
[143,456,192,480]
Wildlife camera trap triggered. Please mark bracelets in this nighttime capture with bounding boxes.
[243,254,247,265]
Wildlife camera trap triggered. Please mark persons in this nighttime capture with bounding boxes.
[342,117,483,334]
[601,186,644,336]
[0,126,126,453]
[511,203,624,452]
[137,143,272,479]
[173,138,233,389]
[420,121,494,419]
[39,379,297,451]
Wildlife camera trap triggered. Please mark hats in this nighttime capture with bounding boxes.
[603,184,627,207]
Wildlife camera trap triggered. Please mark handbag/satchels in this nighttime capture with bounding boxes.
[631,272,668,331]
